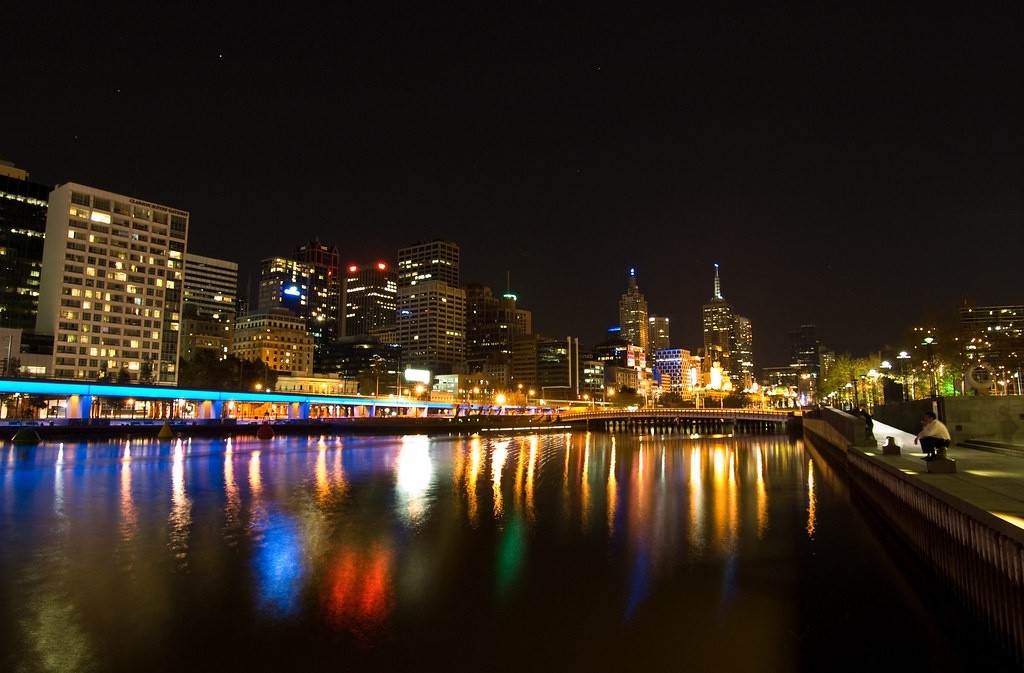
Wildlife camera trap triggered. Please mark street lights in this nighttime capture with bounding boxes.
[897,352,911,401]
[822,360,892,408]
[921,337,938,398]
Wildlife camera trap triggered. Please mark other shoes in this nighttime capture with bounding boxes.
[920,457,930,460]
[926,457,935,460]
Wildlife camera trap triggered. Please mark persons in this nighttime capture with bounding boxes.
[847,404,873,435]
[915,410,952,462]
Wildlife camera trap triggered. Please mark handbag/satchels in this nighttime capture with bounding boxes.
[886,436,896,447]
